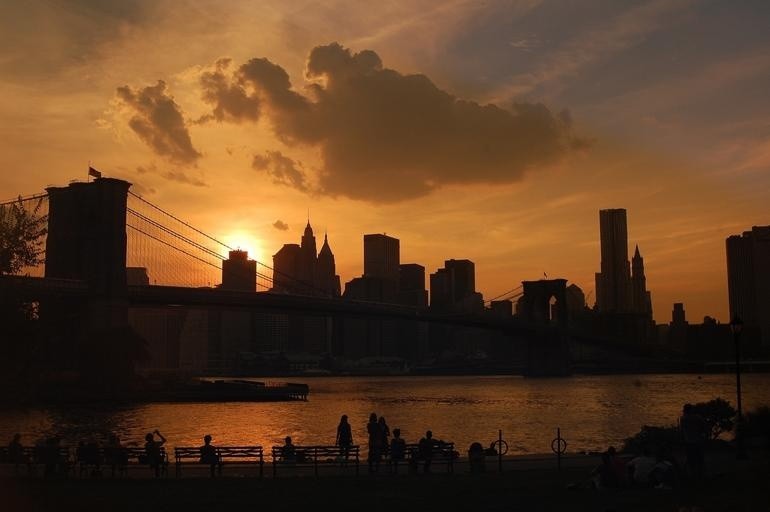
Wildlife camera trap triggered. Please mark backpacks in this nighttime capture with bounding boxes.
[370,429,386,451]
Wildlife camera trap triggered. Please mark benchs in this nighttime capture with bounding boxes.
[367,442,454,478]
[174,444,264,474]
[270,443,362,473]
[3,443,170,479]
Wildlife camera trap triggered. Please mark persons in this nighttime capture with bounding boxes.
[367,413,404,464]
[335,415,353,468]
[281,436,296,462]
[419,431,433,469]
[144,429,167,480]
[200,436,222,477]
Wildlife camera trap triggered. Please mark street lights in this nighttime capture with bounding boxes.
[726,316,744,416]
